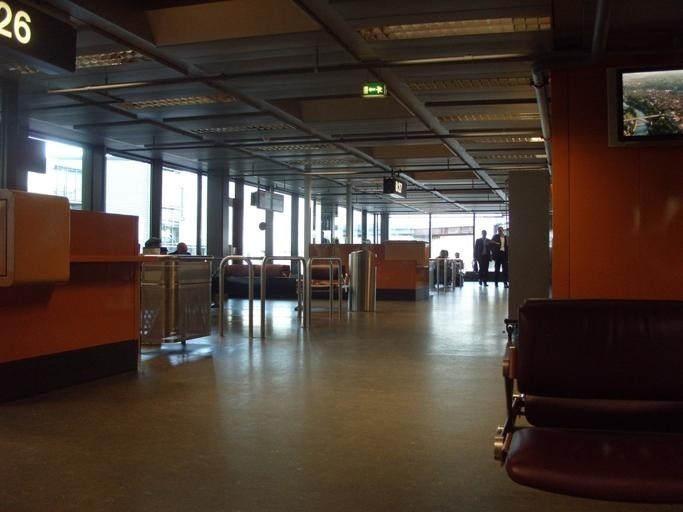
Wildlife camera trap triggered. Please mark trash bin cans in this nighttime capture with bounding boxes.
[348,250,377,311]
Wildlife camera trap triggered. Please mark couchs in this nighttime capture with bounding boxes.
[224,264,297,300]
[306,264,347,300]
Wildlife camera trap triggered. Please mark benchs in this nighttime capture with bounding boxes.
[492,299,683,506]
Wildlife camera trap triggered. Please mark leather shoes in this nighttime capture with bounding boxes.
[479,280,509,287]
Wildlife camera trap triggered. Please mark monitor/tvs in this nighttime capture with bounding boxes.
[272,192,283,212]
[251,189,271,209]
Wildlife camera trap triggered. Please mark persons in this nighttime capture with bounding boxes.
[473,230,494,287]
[434,250,464,287]
[491,226,508,288]
[169,242,191,255]
[321,231,329,243]
[334,238,339,244]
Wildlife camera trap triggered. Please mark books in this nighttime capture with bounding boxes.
[143,247,169,255]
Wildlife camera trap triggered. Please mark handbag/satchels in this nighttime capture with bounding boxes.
[473,261,478,273]
